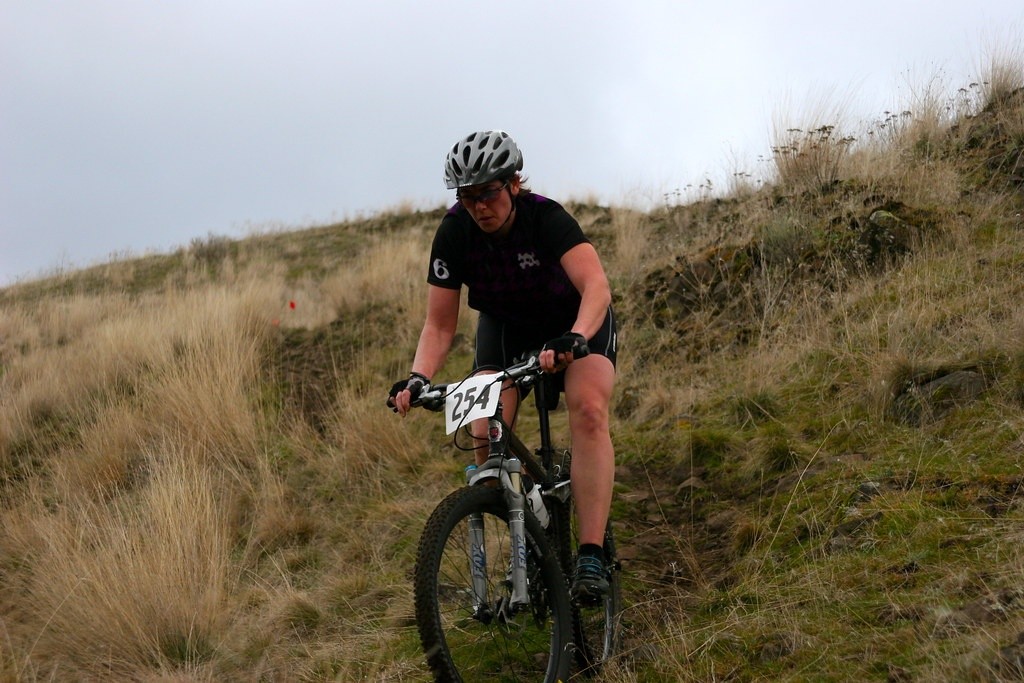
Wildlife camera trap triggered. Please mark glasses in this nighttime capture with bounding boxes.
[456,181,510,207]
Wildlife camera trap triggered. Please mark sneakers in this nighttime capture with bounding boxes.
[507,558,513,584]
[572,556,609,599]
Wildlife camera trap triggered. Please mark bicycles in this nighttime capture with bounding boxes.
[383,342,631,683]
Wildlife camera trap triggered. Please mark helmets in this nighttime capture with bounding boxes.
[444,131,523,190]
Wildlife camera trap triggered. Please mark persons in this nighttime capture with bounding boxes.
[390,130,617,595]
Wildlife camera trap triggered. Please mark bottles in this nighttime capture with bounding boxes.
[516,474,550,529]
